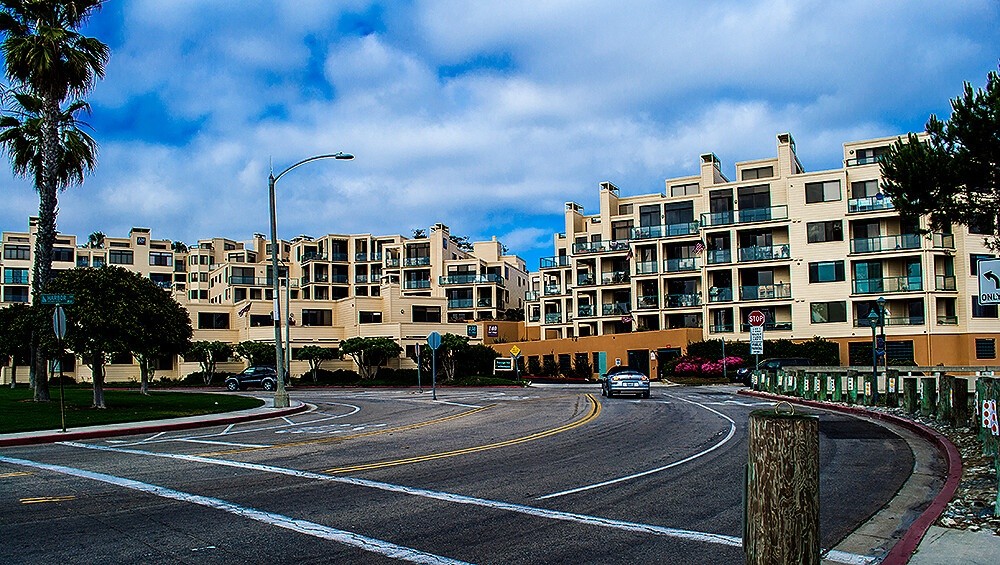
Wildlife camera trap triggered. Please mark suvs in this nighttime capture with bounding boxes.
[224,365,290,392]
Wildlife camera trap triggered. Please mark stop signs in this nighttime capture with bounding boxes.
[748,311,765,326]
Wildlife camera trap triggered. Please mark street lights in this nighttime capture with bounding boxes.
[268,153,358,408]
[869,308,878,374]
[876,296,886,373]
